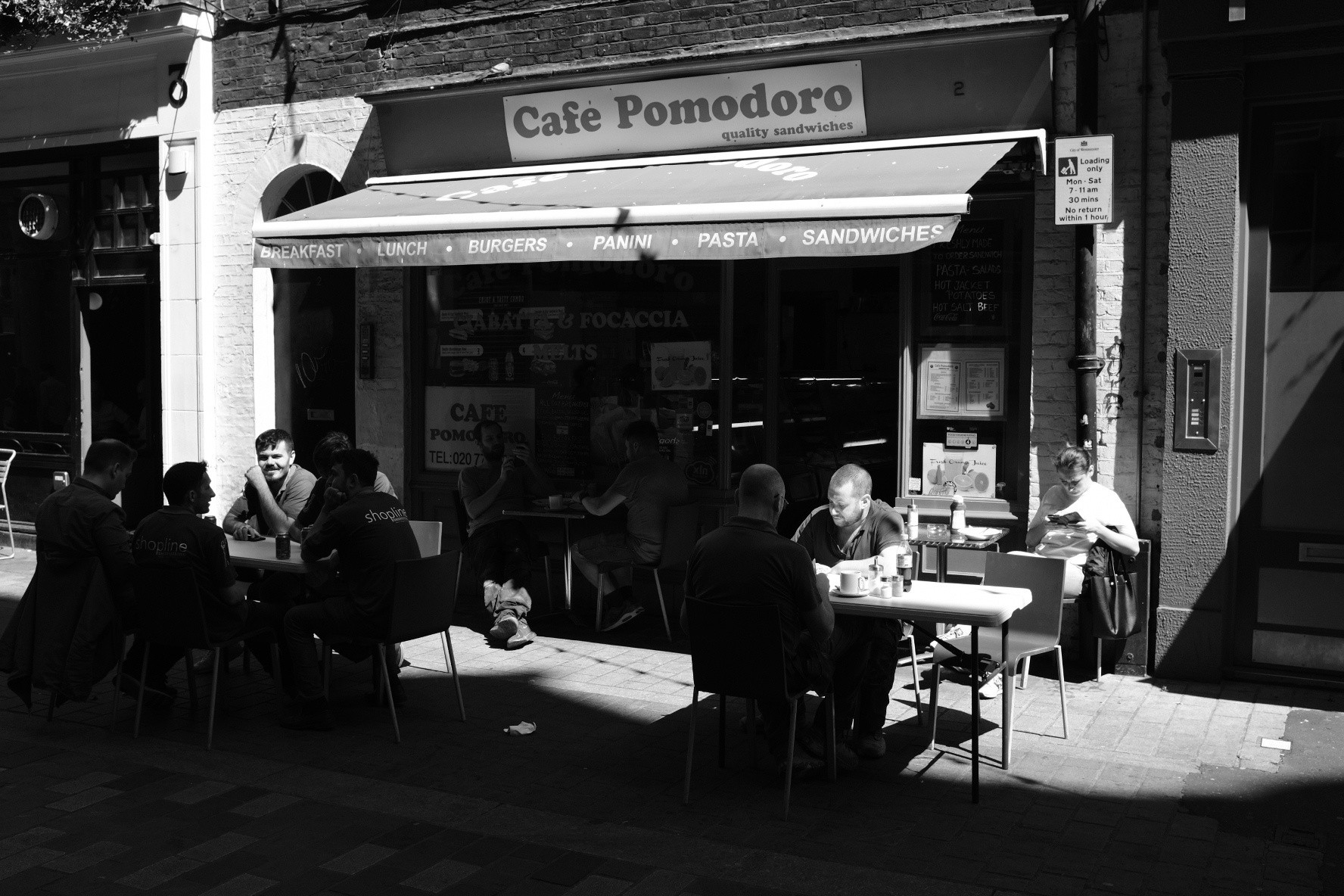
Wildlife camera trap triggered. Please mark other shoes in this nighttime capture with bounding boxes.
[280,709,333,731]
[738,715,765,729]
[600,598,641,632]
[489,612,538,649]
[367,683,408,702]
[120,662,178,704]
[857,732,887,759]
[802,734,859,771]
[776,754,827,785]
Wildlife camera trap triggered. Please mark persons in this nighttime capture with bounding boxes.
[222,428,320,673]
[131,463,322,691]
[290,431,404,669]
[276,449,423,732]
[931,446,1139,698]
[571,419,690,633]
[789,464,913,758]
[685,464,876,774]
[457,420,558,649]
[34,439,177,707]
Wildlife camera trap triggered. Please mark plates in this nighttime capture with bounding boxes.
[570,504,586,511]
[543,505,568,511]
[831,581,874,596]
[958,526,1001,540]
[533,498,573,505]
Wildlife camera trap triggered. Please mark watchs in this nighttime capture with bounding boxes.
[579,492,589,504]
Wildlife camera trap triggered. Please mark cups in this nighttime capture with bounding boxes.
[839,570,866,593]
[927,524,946,533]
[549,494,562,508]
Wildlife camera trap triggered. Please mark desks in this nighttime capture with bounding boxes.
[896,521,1010,668]
[827,577,1033,815]
[224,532,340,675]
[502,504,591,630]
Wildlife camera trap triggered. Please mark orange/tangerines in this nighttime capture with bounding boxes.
[926,469,988,492]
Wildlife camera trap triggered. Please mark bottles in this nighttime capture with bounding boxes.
[896,534,913,592]
[950,494,968,533]
[908,498,919,526]
[868,558,884,597]
[891,574,904,598]
[881,576,892,598]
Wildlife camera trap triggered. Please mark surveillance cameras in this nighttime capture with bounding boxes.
[166,148,186,176]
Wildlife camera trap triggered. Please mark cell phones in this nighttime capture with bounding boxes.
[1047,514,1061,524]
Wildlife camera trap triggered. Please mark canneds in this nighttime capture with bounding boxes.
[275,533,290,559]
[300,527,310,545]
[204,516,216,525]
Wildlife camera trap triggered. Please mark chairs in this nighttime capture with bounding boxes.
[0,487,1105,822]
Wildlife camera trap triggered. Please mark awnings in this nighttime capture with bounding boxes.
[250,137,1017,269]
